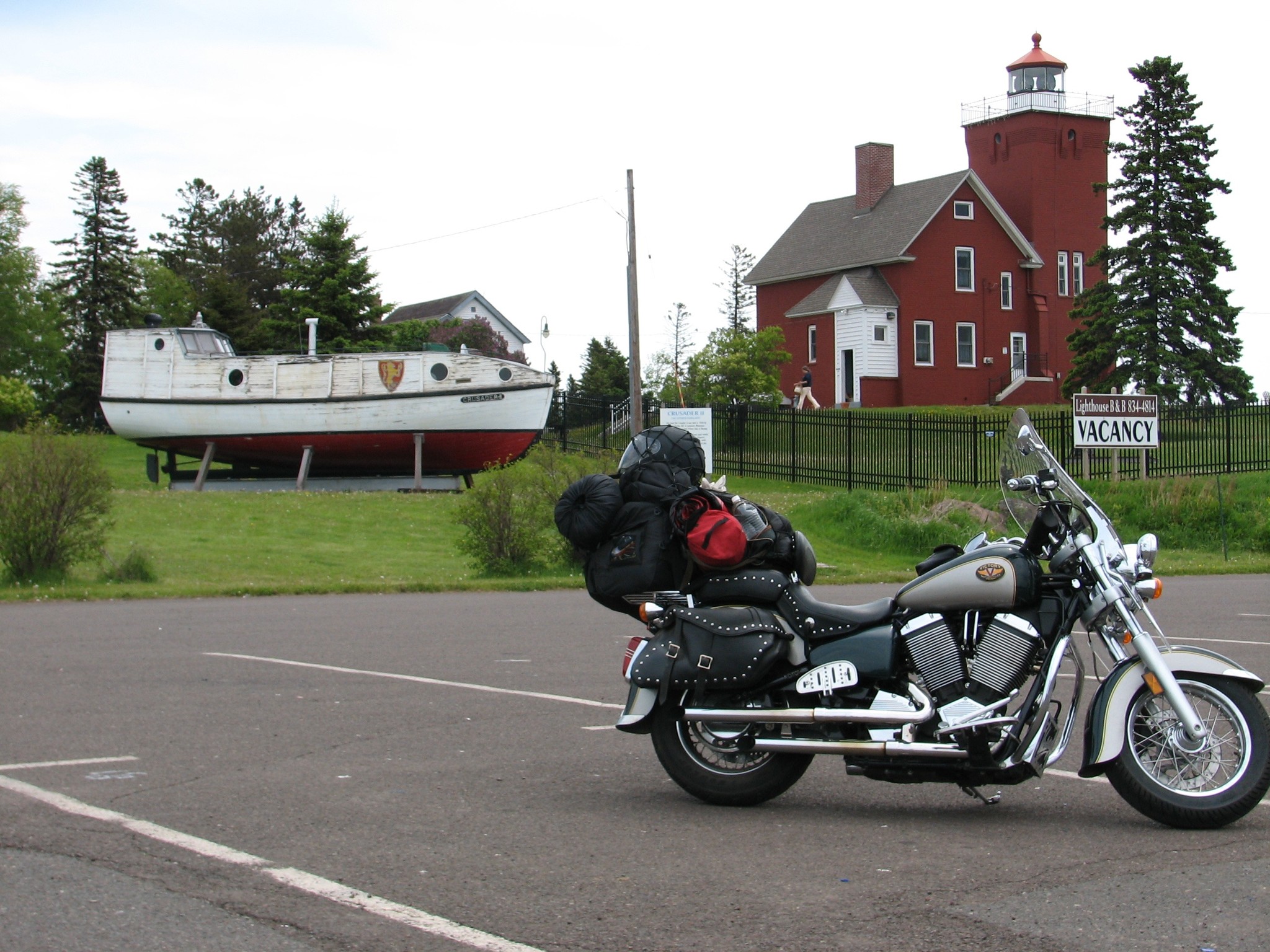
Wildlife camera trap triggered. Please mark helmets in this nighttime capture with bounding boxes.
[623,426,705,485]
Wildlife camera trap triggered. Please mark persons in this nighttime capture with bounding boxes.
[795,366,820,412]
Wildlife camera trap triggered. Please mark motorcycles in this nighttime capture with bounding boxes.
[607,403,1269,832]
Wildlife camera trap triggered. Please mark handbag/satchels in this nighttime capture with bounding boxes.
[555,474,797,692]
[794,383,804,395]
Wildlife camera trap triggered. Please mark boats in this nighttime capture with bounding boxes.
[94,309,557,492]
